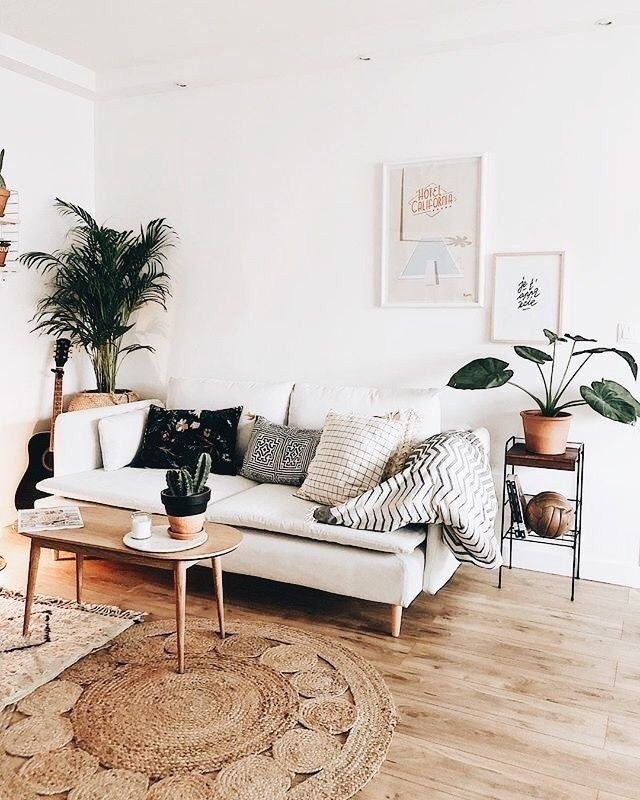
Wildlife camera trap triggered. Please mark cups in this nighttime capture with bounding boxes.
[130,511,151,539]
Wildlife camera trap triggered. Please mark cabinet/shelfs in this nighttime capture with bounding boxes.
[497,436,585,601]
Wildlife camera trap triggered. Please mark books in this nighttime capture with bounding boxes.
[18,505,84,534]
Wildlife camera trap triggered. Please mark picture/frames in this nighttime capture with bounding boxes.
[490,250,564,345]
[379,151,487,308]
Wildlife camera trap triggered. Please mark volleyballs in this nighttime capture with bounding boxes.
[526,491,575,539]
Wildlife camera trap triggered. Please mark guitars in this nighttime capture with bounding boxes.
[14,337,75,512]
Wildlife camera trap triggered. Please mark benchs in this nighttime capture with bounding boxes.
[35,398,491,636]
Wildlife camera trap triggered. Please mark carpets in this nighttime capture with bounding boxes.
[0,586,149,710]
[0,617,398,800]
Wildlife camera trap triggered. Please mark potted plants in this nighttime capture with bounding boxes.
[15,197,181,413]
[447,330,640,455]
[160,453,211,540]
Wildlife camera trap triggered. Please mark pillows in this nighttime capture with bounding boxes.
[238,414,322,486]
[98,407,150,470]
[375,408,417,485]
[292,409,403,508]
[129,403,243,476]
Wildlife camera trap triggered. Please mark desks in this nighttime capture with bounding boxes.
[12,506,243,674]
